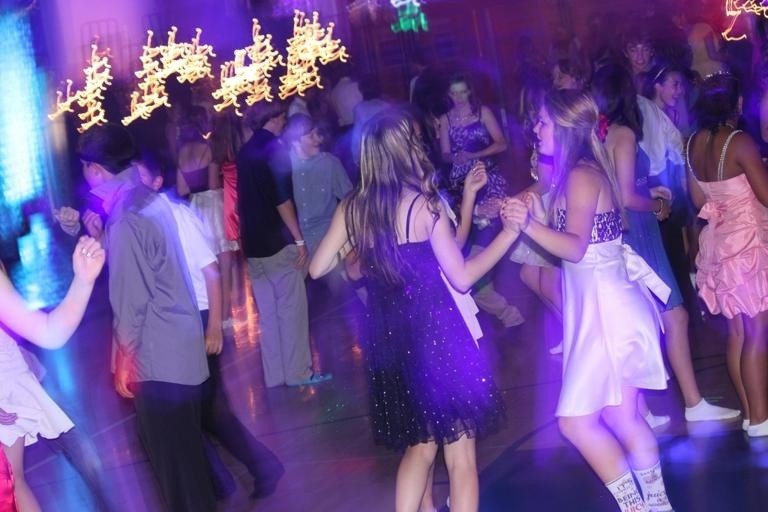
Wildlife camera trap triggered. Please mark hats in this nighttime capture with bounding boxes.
[77,123,134,164]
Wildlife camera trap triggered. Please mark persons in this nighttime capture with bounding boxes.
[1,1,768,512]
